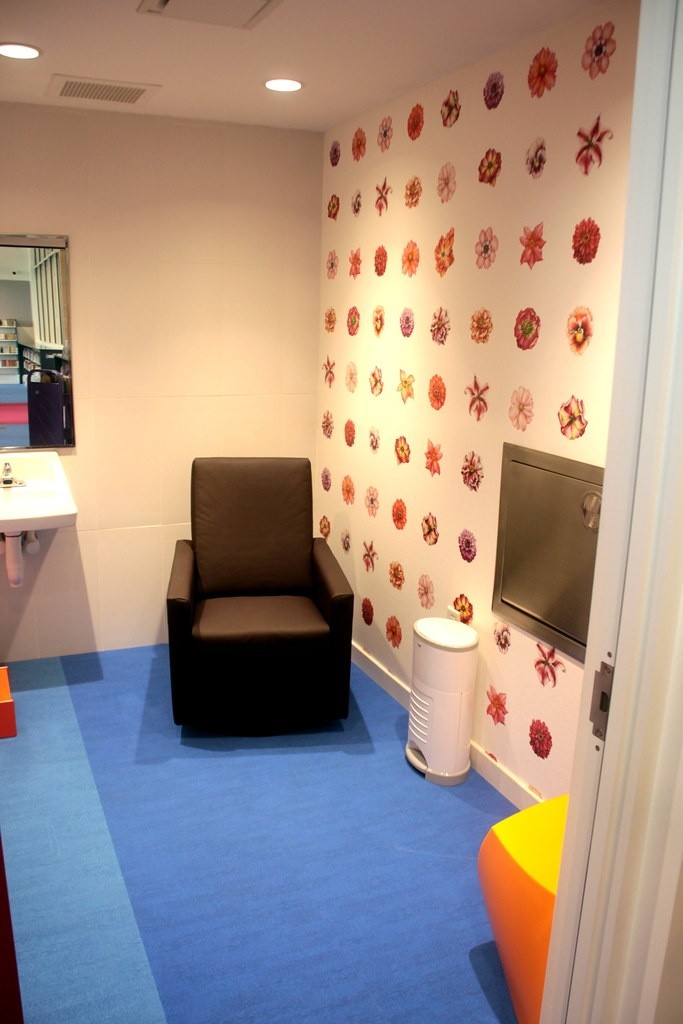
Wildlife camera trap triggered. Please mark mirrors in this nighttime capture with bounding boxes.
[0,234,76,453]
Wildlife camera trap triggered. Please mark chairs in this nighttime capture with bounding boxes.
[164,454,355,738]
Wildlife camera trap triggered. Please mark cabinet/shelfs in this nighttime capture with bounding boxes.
[0,319,19,375]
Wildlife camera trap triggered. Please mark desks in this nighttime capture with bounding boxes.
[16,342,62,384]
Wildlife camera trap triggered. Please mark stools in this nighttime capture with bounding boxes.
[476,792,571,1024]
